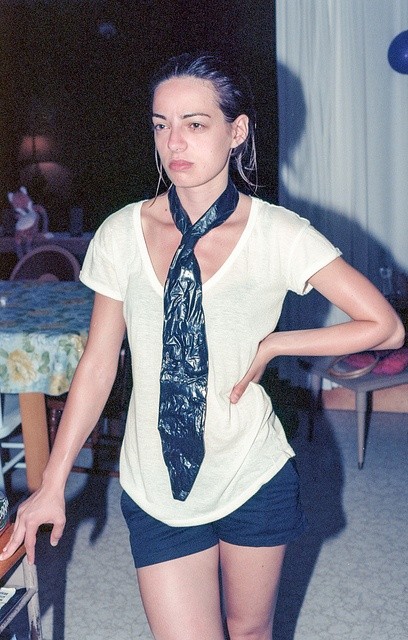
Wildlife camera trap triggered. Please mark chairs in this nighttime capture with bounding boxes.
[44,372,133,478]
[9,246,81,282]
[0,394,28,500]
[297,274,407,470]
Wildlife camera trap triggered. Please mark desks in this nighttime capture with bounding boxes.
[0,523,43,640]
[0,279,125,531]
[0,232,93,280]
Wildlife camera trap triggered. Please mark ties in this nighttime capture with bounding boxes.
[158,176,238,500]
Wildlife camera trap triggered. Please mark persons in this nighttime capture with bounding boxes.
[0,52,406,640]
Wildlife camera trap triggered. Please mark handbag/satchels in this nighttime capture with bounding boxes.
[328,348,407,381]
